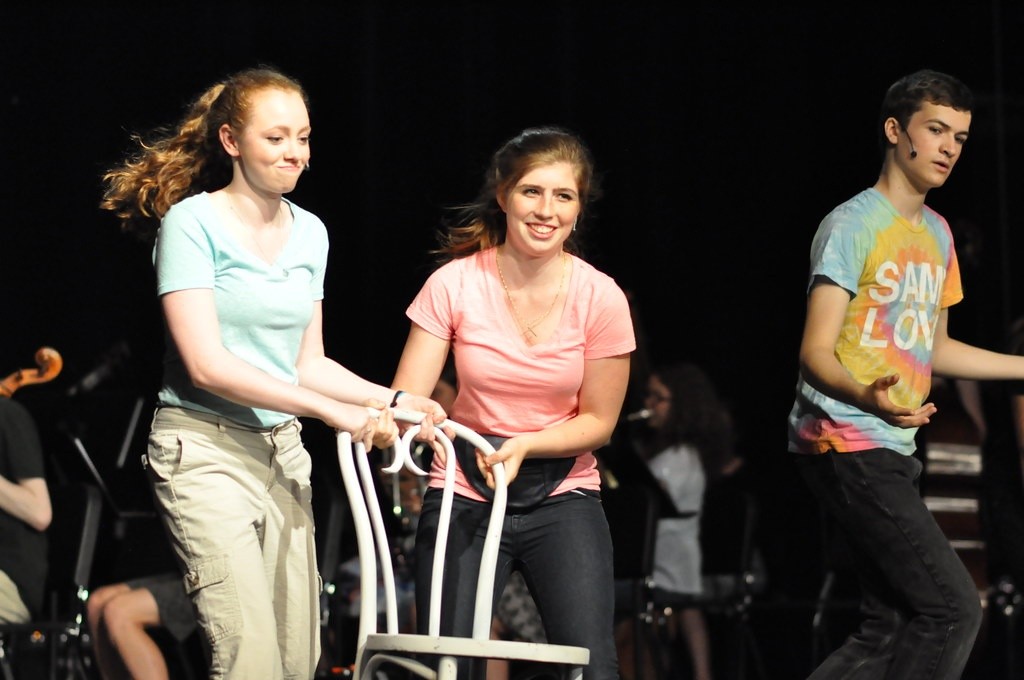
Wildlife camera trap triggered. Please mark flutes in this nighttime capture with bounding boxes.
[627,408,653,422]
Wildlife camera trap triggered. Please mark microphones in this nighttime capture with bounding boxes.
[895,116,917,158]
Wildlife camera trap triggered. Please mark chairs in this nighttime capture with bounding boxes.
[628,487,767,679]
[336,405,591,680]
[0,479,102,680]
[175,498,344,680]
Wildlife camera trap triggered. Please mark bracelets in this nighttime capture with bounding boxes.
[391,389,404,409]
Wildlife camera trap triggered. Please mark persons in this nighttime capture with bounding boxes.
[388,128,636,680]
[788,69,1022,679]
[0,320,757,680]
[99,67,457,680]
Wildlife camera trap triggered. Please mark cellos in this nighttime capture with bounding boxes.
[0,347,64,399]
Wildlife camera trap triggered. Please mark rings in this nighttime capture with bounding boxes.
[361,427,372,435]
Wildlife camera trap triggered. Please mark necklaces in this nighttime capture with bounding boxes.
[496,244,568,339]
[225,184,286,267]
